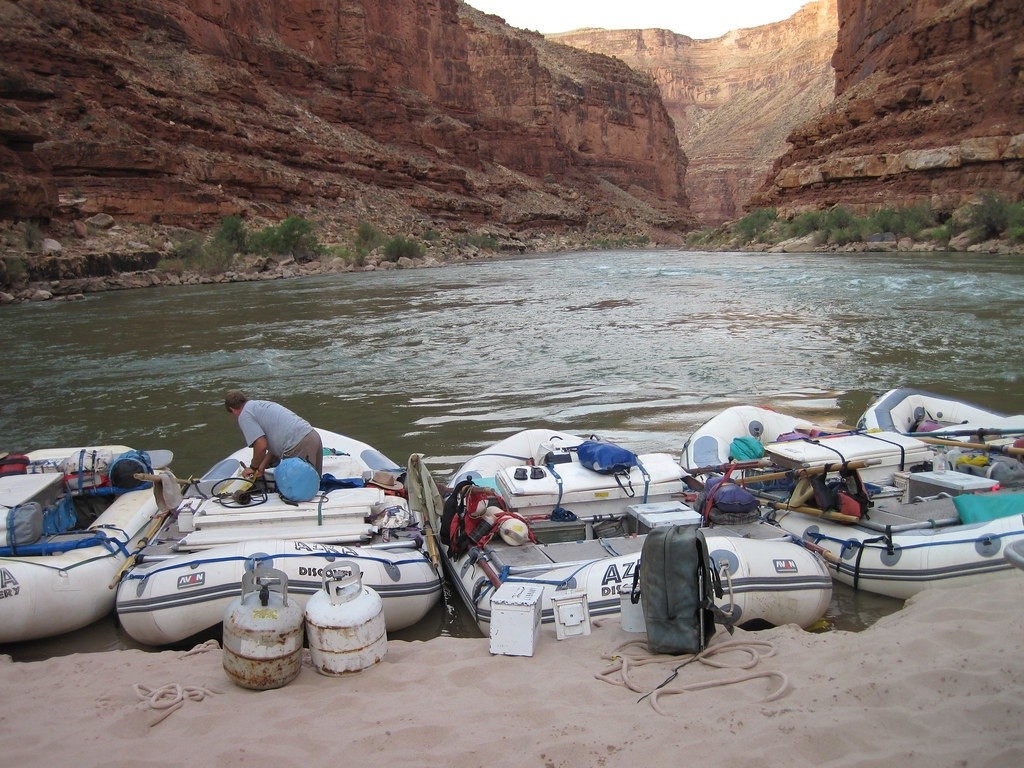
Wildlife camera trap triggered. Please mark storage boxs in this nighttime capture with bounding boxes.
[528,515,586,546]
[177,497,201,532]
[26,458,63,475]
[629,500,703,536]
[907,469,999,504]
[490,577,643,654]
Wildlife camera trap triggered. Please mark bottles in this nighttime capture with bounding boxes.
[933,445,946,475]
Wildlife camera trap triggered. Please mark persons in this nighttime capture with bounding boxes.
[225,390,323,476]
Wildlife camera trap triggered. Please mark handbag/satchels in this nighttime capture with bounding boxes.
[631,525,723,654]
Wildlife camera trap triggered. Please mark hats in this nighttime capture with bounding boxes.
[369,470,404,490]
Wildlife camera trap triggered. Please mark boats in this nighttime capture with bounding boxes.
[678,405,1024,602]
[860,386,1024,490]
[0,445,180,643]
[115,426,446,644]
[436,430,833,637]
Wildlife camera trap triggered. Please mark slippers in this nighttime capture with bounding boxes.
[530,467,544,479]
[514,468,528,480]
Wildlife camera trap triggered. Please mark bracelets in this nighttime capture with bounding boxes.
[249,465,258,470]
